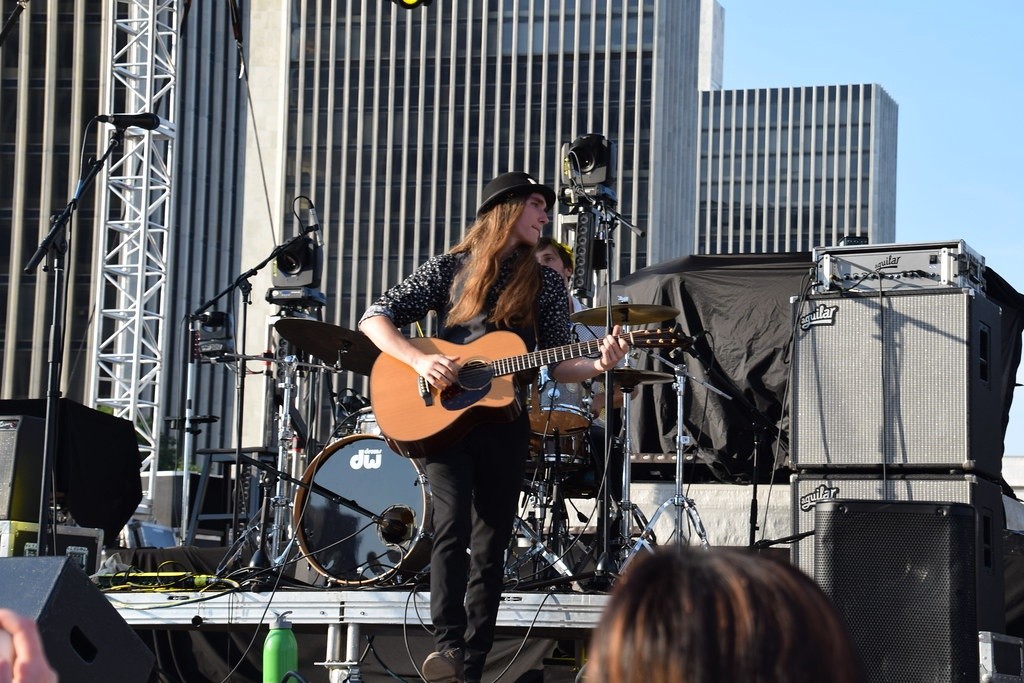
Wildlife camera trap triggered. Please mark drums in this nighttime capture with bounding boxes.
[521,367,597,437]
[293,437,433,587]
[524,432,595,471]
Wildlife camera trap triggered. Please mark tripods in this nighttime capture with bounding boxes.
[503,186,733,593]
[213,349,342,572]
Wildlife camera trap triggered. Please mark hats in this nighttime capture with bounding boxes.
[477,171,556,216]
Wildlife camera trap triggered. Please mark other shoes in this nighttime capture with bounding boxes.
[422,647,464,683]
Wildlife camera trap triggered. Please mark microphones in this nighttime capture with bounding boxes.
[95,113,160,130]
[309,200,324,247]
[381,520,408,537]
[351,389,370,407]
[566,153,577,189]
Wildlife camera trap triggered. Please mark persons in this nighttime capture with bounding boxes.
[357,171,631,683]
[527,236,639,593]
[0,607,60,683]
[586,544,873,683]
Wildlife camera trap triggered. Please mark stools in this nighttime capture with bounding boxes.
[186,446,279,547]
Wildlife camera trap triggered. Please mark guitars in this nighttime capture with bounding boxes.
[369,324,702,460]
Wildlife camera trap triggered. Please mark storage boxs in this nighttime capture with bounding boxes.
[0,521,103,578]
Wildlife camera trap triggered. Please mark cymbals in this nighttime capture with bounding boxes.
[273,317,380,374]
[592,366,679,390]
[567,304,681,327]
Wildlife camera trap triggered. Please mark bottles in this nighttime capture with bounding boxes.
[262,611,299,683]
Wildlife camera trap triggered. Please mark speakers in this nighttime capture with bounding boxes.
[790,288,1005,683]
[0,397,143,547]
[0,556,155,683]
